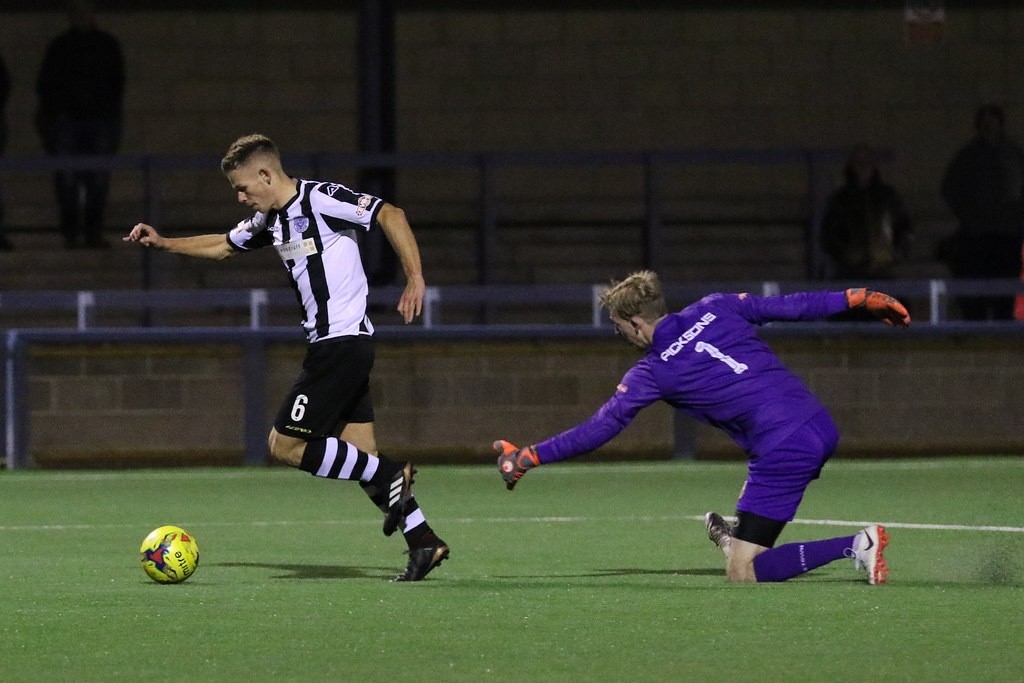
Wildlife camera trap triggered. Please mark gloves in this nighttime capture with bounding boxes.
[845,287,911,330]
[493,440,540,491]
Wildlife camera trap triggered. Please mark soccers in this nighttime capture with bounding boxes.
[140,525,199,584]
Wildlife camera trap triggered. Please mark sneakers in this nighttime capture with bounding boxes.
[393,529,451,581]
[382,461,417,537]
[704,512,733,557]
[843,524,892,586]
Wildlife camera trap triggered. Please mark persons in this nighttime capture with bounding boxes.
[826,146,912,322]
[493,270,911,583]
[34,0,126,249]
[121,133,449,581]
[933,103,1023,314]
